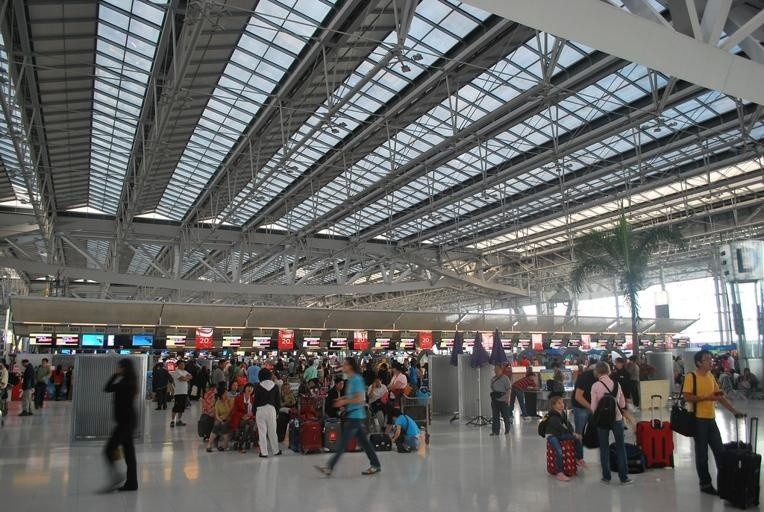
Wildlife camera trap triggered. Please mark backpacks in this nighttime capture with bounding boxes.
[582,412,600,448]
[539,413,563,437]
[592,378,617,429]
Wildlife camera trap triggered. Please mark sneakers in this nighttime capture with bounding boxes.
[170,422,174,427]
[620,478,633,484]
[556,472,570,480]
[175,420,186,426]
[600,477,608,483]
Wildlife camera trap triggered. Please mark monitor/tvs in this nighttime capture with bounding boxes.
[330,337,347,348]
[55,333,79,347]
[132,335,154,346]
[252,337,272,347]
[29,332,53,346]
[399,338,415,349]
[440,338,691,349]
[165,335,187,346]
[302,337,321,348]
[107,334,114,347]
[81,333,106,348]
[222,336,241,348]
[374,337,391,348]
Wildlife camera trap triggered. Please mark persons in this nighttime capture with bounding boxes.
[153,354,427,477]
[490,351,759,494]
[102,358,139,492]
[0,354,73,426]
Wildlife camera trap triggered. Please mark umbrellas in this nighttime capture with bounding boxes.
[489,328,507,366]
[451,332,464,368]
[469,331,489,426]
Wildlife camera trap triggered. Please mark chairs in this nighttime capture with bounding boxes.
[716,373,763,403]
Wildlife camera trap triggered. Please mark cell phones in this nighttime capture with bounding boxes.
[714,391,724,396]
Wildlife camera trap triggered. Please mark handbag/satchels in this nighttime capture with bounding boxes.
[369,398,382,416]
[609,442,645,473]
[370,433,391,451]
[236,375,248,386]
[670,372,696,437]
[490,392,503,400]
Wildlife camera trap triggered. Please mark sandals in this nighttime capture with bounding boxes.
[361,465,381,475]
[313,464,331,476]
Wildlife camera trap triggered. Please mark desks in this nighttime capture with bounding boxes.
[524,387,574,419]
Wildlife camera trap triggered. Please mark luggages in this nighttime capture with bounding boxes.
[636,395,674,468]
[170,384,192,409]
[723,414,747,450]
[546,439,576,477]
[287,416,363,454]
[729,417,761,509]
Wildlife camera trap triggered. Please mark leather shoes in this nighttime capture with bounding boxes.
[275,450,281,455]
[118,483,136,490]
[19,411,29,416]
[207,441,258,453]
[700,486,717,495]
[258,453,267,458]
